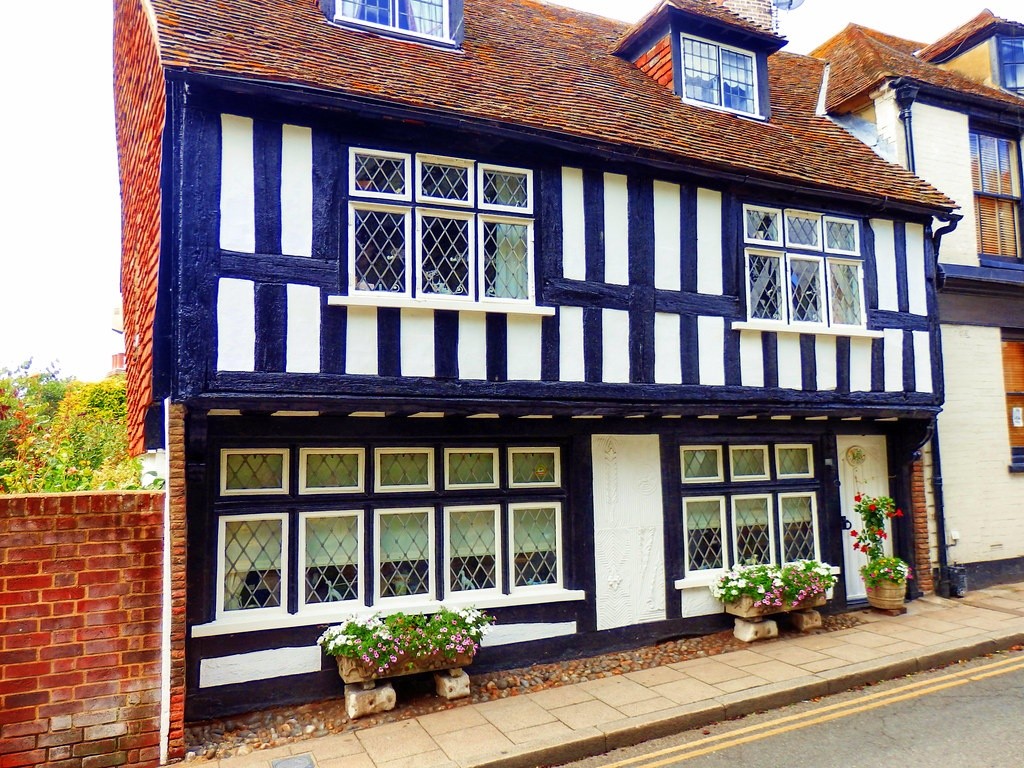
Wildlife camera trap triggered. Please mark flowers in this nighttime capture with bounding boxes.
[851,490,914,592]
[316,605,498,673]
[708,554,839,620]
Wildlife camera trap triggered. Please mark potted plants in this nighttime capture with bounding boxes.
[865,579,907,609]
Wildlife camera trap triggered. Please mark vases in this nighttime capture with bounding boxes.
[726,591,827,622]
[337,646,472,690]
